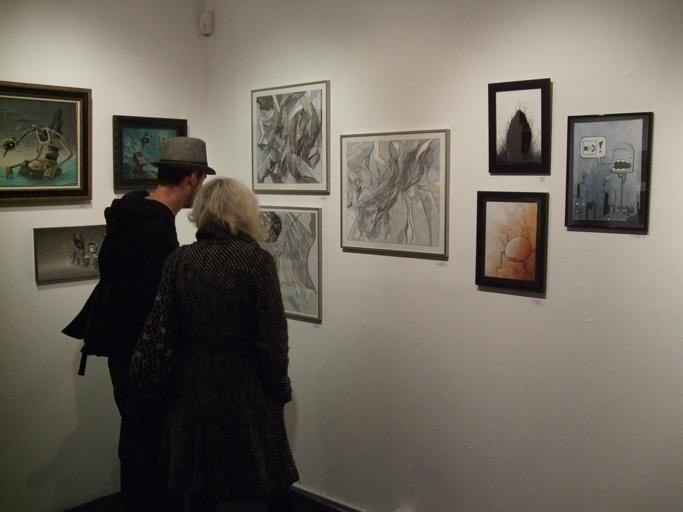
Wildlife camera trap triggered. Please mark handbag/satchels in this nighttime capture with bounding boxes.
[128,245,189,402]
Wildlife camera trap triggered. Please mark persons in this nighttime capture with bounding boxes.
[96,137,217,509]
[128,178,300,511]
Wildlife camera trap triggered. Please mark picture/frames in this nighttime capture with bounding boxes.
[564,111,652,235]
[473,189,547,298]
[112,115,185,192]
[340,130,447,263]
[31,224,111,287]
[252,79,329,195]
[254,205,322,322]
[488,78,550,175]
[0,79,91,206]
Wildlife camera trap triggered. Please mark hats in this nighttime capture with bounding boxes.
[149,137,216,175]
